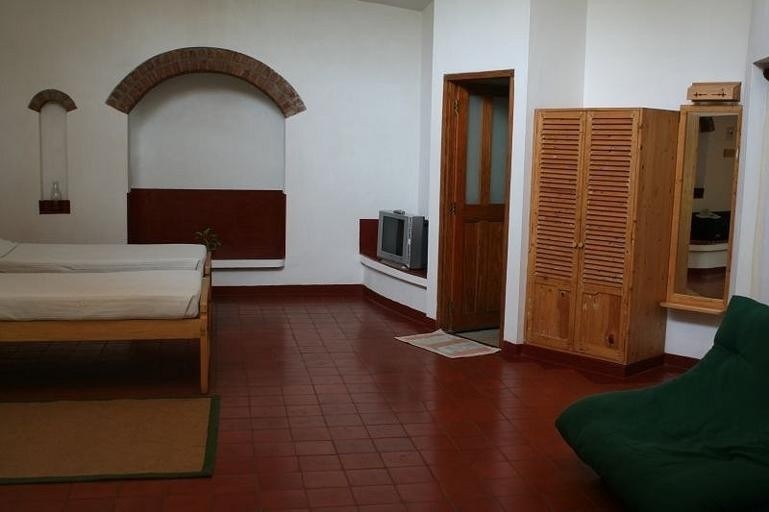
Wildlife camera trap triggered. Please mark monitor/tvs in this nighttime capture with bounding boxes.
[377,210,428,272]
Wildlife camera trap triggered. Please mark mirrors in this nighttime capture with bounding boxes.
[661,102,750,316]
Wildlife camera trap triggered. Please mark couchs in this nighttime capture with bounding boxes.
[555,291,768,512]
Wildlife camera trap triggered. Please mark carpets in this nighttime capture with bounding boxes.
[392,326,502,360]
[1,389,219,483]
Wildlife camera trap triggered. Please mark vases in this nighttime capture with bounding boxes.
[50,180,62,200]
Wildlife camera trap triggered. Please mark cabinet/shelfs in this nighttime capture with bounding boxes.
[518,107,683,377]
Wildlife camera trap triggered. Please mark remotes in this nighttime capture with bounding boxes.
[393,209,405,214]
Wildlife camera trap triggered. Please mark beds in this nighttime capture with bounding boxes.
[0,239,213,276]
[0,270,212,393]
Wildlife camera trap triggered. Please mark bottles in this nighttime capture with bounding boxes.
[49,182,61,201]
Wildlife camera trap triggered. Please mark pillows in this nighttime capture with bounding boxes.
[0,237,18,258]
